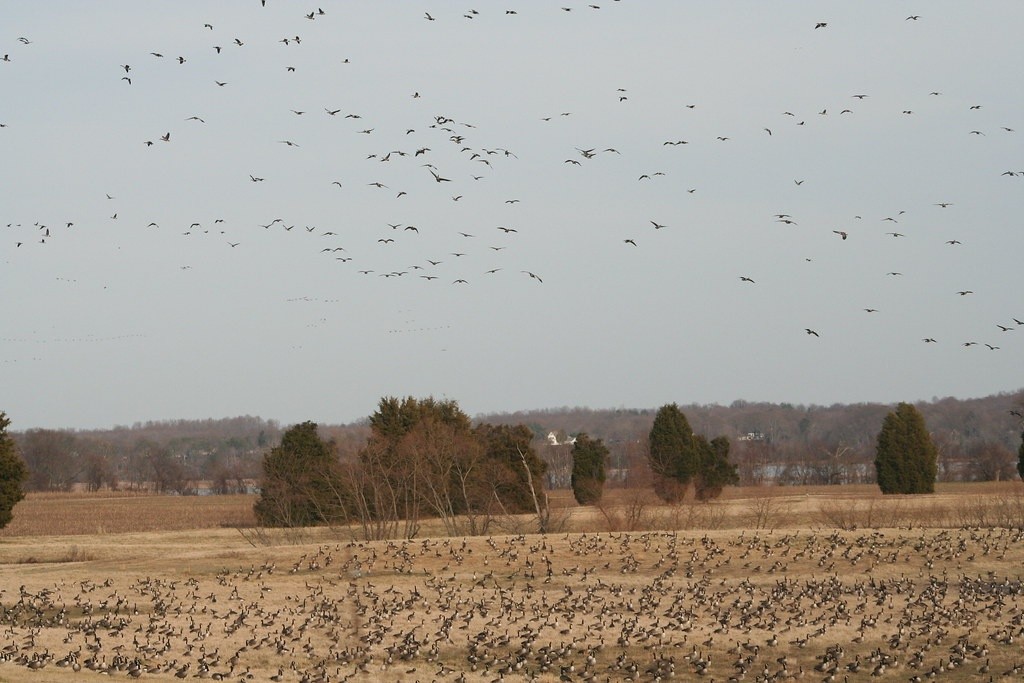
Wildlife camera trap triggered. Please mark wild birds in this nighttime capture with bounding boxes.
[2,1,1022,363]
[0,506,1023,680]
